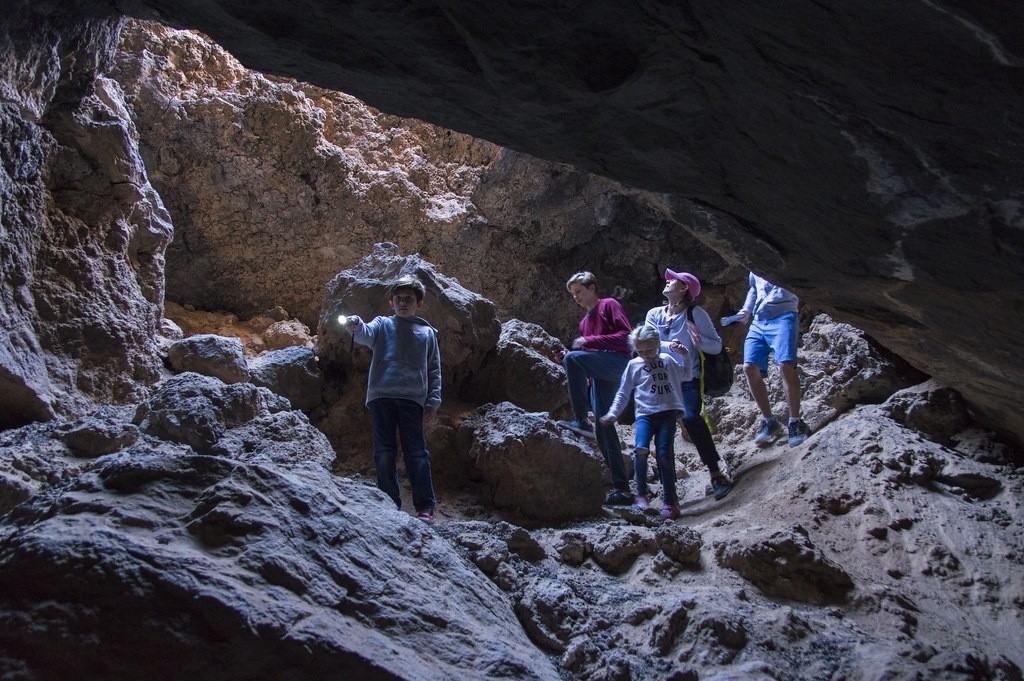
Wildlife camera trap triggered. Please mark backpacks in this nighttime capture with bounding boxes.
[687,304,734,398]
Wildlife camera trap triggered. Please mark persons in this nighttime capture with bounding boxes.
[737,271,808,448]
[600,326,693,519]
[345,277,442,524]
[560,271,633,506]
[644,268,729,500]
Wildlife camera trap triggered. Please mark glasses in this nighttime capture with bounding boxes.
[662,317,673,335]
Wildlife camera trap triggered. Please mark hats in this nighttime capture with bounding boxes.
[665,268,701,298]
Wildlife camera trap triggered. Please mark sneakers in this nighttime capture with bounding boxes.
[636,497,650,512]
[660,505,681,519]
[602,491,638,510]
[416,508,435,524]
[754,419,784,448]
[711,472,732,500]
[788,419,809,448]
[557,420,597,439]
